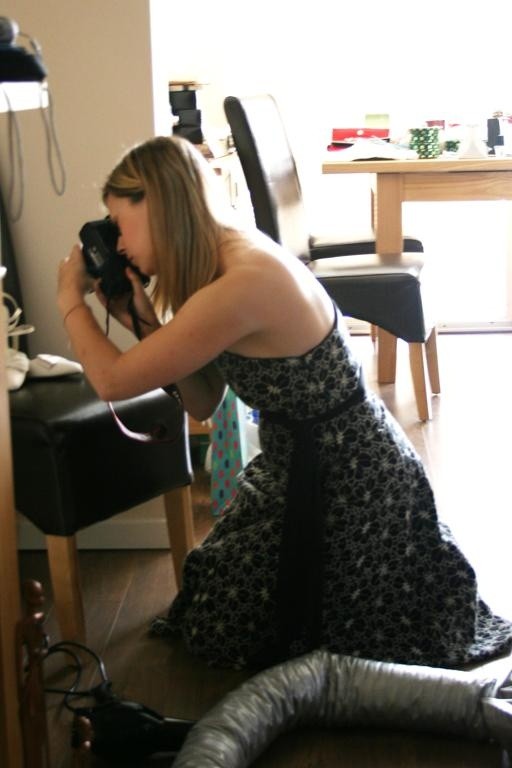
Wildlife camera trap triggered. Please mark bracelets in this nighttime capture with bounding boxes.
[62,302,85,324]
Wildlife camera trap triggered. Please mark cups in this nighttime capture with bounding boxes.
[493,144,504,157]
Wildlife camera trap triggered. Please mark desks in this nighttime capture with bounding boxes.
[322,158,512,386]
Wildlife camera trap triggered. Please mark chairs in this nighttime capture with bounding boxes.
[223,96,441,421]
[309,236,423,351]
[2,196,197,666]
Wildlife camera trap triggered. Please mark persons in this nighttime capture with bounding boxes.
[56,135,511,672]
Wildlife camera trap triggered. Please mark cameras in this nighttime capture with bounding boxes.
[72,216,151,300]
